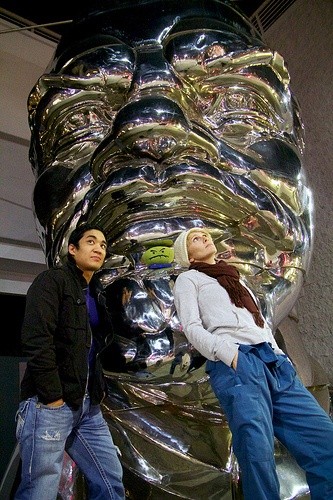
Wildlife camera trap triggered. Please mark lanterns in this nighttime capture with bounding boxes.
[141,246,173,268]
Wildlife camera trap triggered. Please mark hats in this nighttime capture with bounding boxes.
[174,228,213,268]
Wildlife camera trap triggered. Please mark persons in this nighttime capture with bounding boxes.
[12,224,124,500]
[171,228,333,500]
[90,267,207,377]
